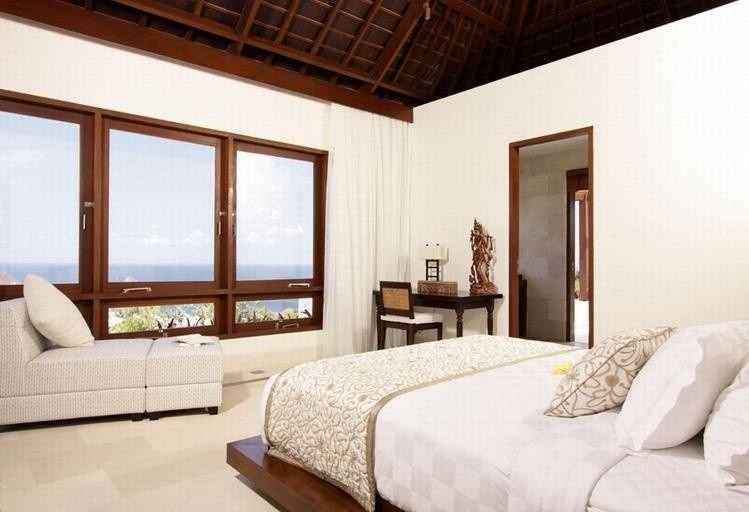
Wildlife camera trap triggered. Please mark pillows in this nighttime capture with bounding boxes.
[544,319,748,486]
[23,273,95,348]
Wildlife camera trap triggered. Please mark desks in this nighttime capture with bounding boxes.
[373,288,503,350]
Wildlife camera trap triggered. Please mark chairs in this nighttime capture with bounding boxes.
[379,280,443,349]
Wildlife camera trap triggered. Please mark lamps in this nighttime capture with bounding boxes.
[418,242,448,280]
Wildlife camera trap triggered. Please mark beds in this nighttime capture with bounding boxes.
[260,334,749,512]
[1,296,224,433]
